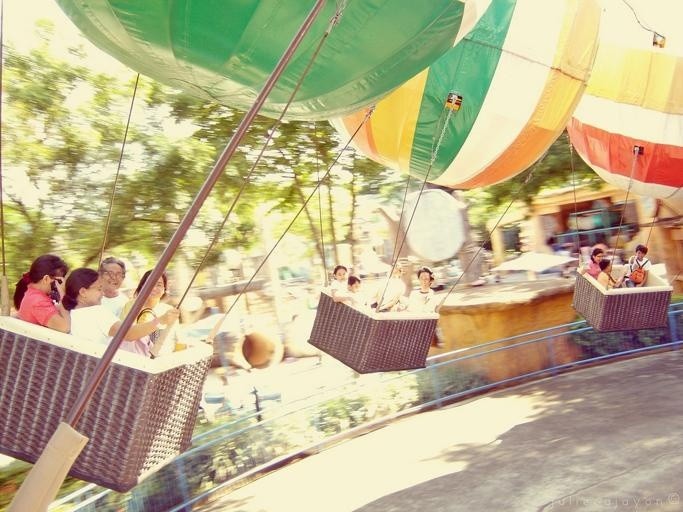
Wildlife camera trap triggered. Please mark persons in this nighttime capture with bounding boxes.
[624,244,651,288]
[581,248,603,280]
[597,259,616,289]
[397,267,436,313]
[330,265,348,295]
[61,268,179,353]
[121,270,177,350]
[332,276,366,305]
[97,257,129,316]
[12,254,69,333]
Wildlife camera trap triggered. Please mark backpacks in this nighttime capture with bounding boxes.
[629,261,648,284]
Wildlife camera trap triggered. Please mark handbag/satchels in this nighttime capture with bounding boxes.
[138,308,176,356]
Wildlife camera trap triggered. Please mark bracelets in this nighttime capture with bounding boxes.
[152,318,163,328]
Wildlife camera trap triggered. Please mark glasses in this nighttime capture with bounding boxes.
[102,271,125,279]
